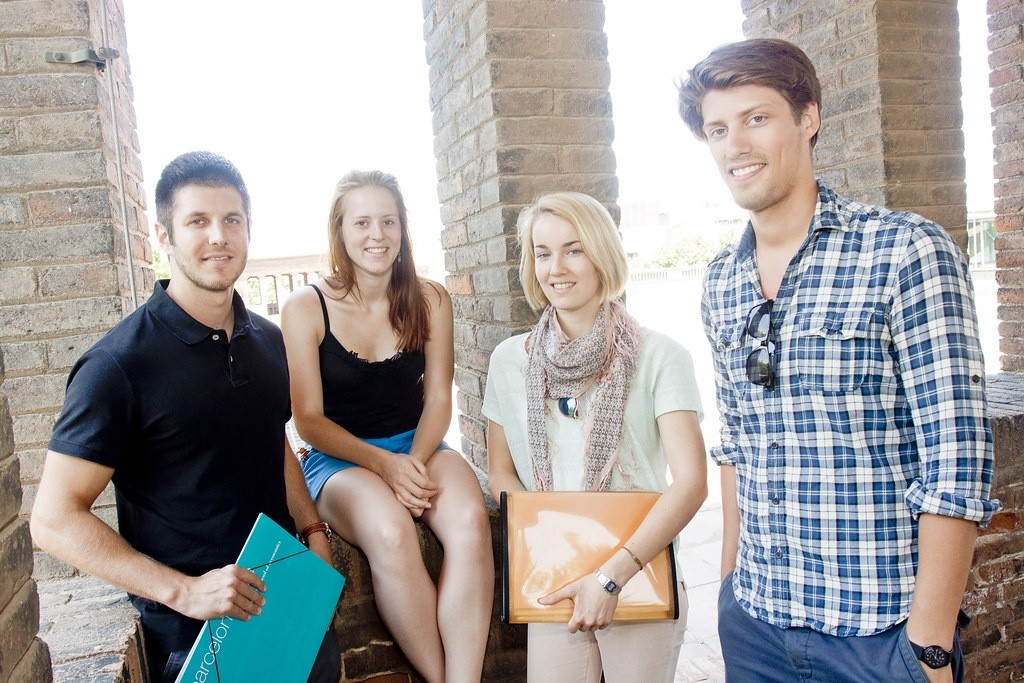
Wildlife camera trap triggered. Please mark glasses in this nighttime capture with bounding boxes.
[744,298,777,388]
[558,397,579,419]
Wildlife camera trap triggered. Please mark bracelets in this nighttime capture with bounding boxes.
[621,546,643,571]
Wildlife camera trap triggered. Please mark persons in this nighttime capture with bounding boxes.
[479,193,709,683]
[29,152,333,683]
[279,171,494,683]
[677,39,1002,683]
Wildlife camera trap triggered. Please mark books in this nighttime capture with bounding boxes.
[175,513,345,683]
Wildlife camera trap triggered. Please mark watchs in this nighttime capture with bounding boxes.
[595,568,622,595]
[909,639,954,670]
[299,522,332,547]
